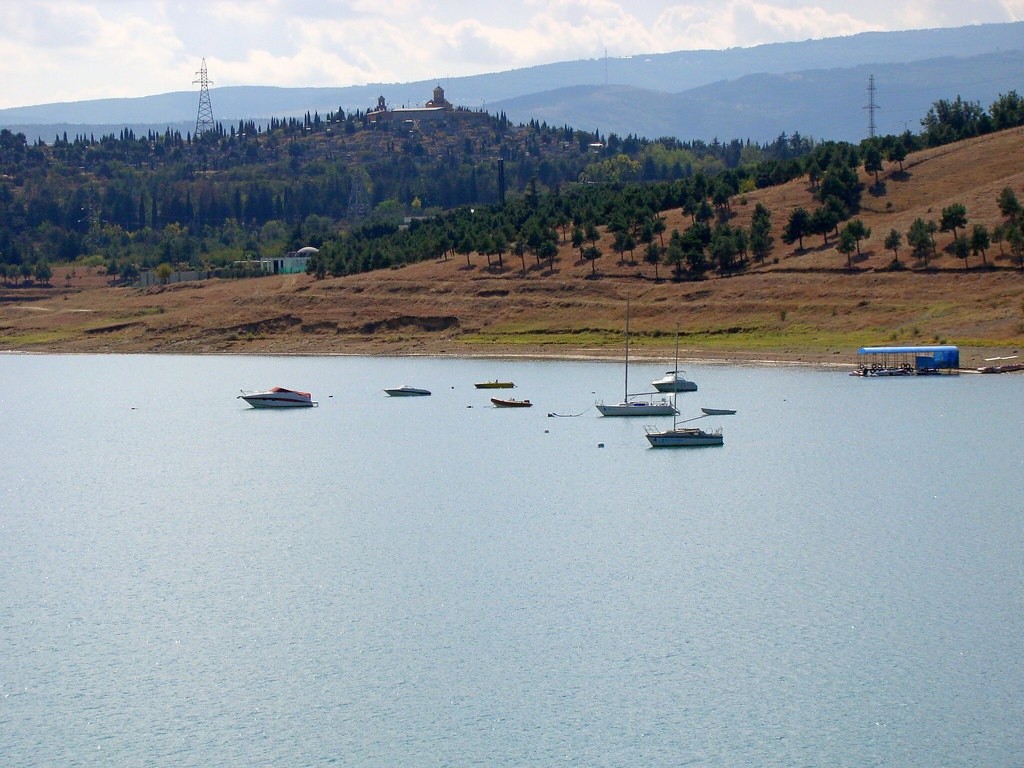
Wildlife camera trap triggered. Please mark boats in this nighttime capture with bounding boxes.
[976,362,1024,374]
[237,387,320,408]
[700,407,737,415]
[491,398,533,408]
[651,371,698,392]
[848,346,959,378]
[383,384,431,397]
[474,379,518,388]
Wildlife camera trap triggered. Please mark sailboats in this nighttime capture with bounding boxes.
[593,289,680,416]
[642,322,724,446]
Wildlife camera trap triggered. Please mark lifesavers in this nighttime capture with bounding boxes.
[934,368,939,372]
[901,363,914,372]
[860,364,864,369]
[870,363,882,374]
[924,368,928,372]
